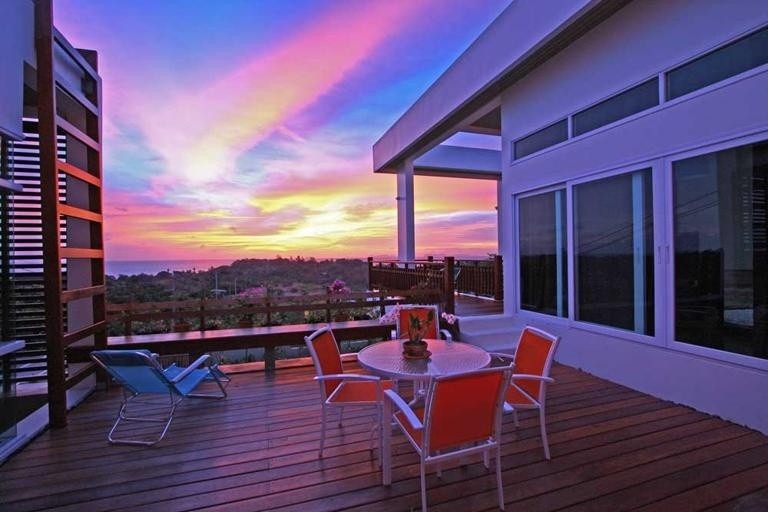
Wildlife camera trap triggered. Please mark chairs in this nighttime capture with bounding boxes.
[89,348,231,447]
